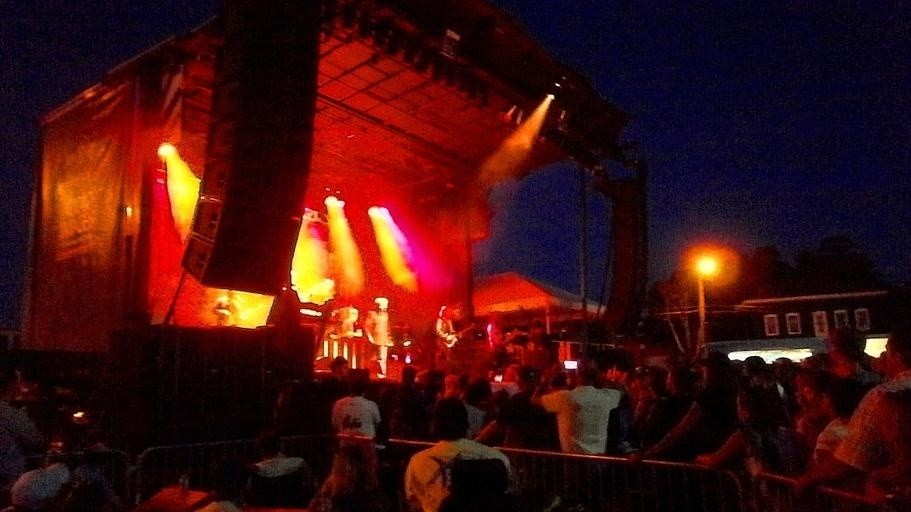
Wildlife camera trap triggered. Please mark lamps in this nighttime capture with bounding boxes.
[320,1,491,110]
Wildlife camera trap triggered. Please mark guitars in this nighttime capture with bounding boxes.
[443,323,474,348]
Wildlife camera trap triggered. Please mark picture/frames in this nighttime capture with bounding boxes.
[833,309,849,329]
[853,307,871,331]
[763,313,780,337]
[812,311,829,338]
[785,312,803,335]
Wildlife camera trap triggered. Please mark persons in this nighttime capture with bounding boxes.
[1,316,911,511]
[364,296,394,381]
[435,304,459,366]
[212,288,248,327]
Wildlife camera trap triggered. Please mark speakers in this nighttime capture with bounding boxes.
[597,178,648,339]
[178,1,318,297]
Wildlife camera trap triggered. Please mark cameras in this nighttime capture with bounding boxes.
[564,360,578,369]
